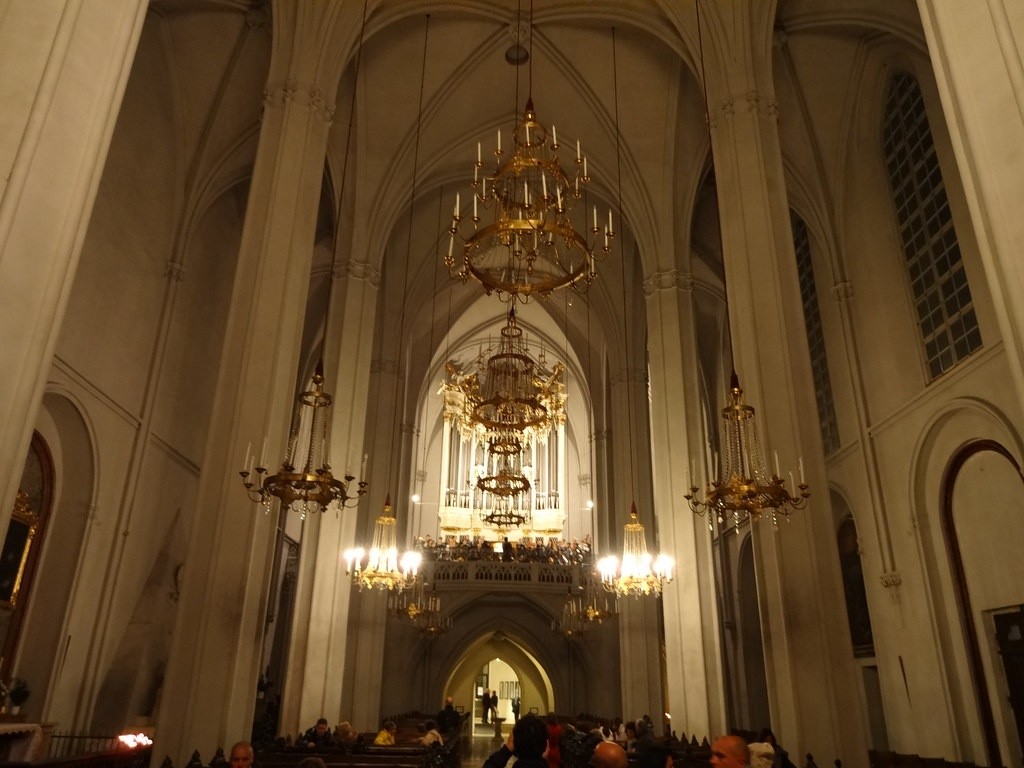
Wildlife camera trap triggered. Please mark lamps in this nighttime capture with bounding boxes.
[341,11,424,592]
[684,1,810,538]
[445,2,614,299]
[594,20,674,599]
[240,0,367,521]
[443,264,572,539]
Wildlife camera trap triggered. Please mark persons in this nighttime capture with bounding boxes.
[300,718,335,747]
[419,720,443,746]
[373,720,398,745]
[709,735,752,768]
[336,721,366,749]
[511,697,520,723]
[299,757,326,768]
[543,711,689,768]
[481,714,552,768]
[758,728,778,747]
[481,690,491,724]
[415,534,592,583]
[228,740,254,768]
[490,691,498,723]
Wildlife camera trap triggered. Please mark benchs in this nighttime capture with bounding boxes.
[255,709,470,768]
